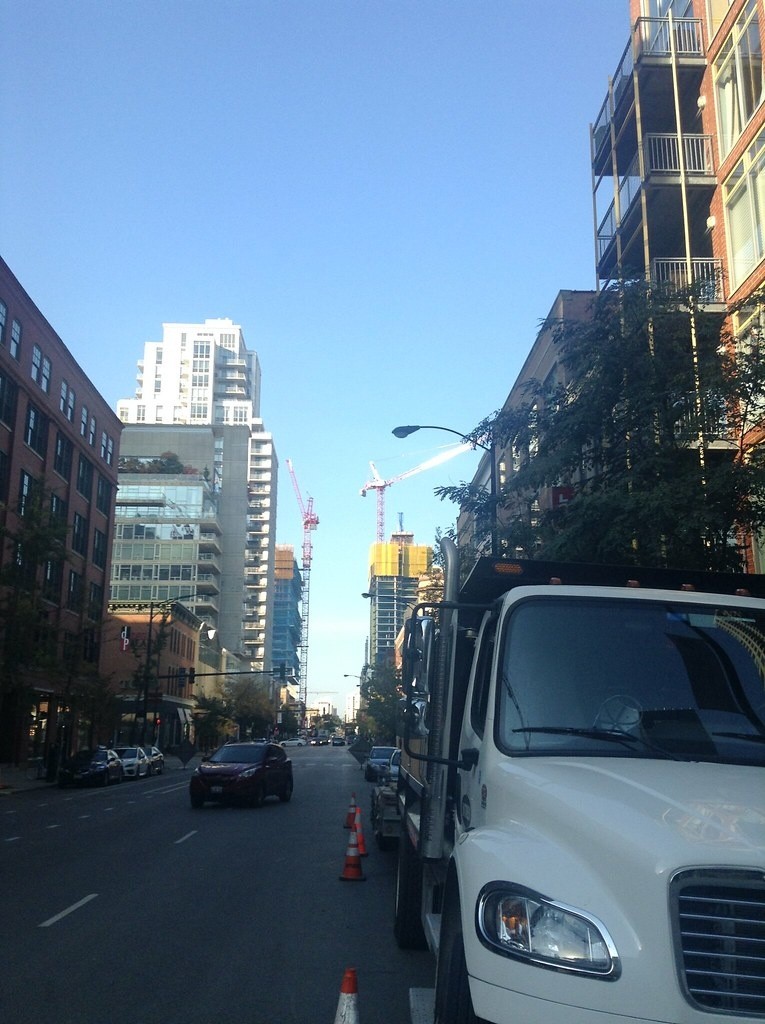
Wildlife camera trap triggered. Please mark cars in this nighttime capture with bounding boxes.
[280,732,357,748]
[366,744,405,788]
[112,747,151,778]
[138,745,164,776]
[62,746,123,785]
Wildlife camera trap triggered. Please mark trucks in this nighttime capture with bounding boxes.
[371,527,764,1024]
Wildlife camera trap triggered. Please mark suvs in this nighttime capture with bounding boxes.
[190,742,293,810]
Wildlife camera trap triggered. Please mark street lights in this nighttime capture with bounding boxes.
[391,423,503,562]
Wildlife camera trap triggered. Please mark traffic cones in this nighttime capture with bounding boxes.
[349,807,369,858]
[342,792,355,829]
[338,822,367,882]
[330,967,361,1024]
